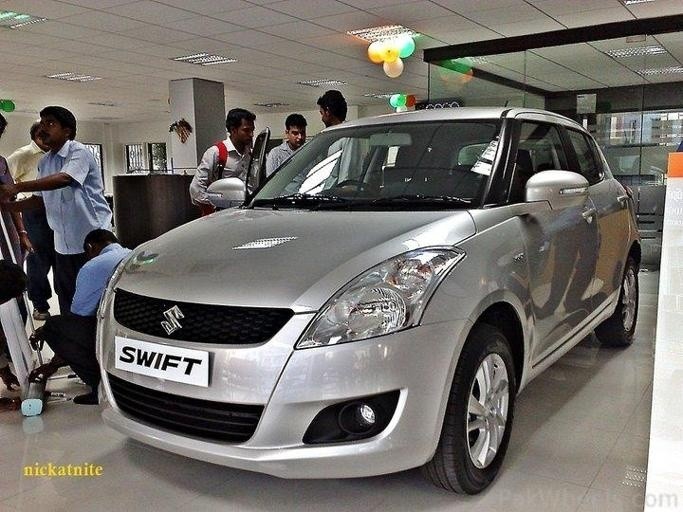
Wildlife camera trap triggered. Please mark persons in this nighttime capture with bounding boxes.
[5,119,51,320]
[188,107,257,218]
[0,113,35,362]
[317,88,362,187]
[0,260,28,412]
[267,112,318,199]
[1,105,113,315]
[28,227,134,405]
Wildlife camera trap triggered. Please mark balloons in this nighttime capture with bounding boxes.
[389,92,417,114]
[367,35,415,79]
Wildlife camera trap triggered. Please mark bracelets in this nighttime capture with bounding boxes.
[17,228,29,237]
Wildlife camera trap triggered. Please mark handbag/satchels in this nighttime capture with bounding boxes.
[0,259,31,305]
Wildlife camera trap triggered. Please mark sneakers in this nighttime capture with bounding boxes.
[0,364,20,392]
[32,309,50,320]
[72,392,99,405]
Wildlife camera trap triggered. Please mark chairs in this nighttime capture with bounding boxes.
[376,141,566,201]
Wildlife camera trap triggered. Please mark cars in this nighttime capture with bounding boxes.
[94,105,642,496]
